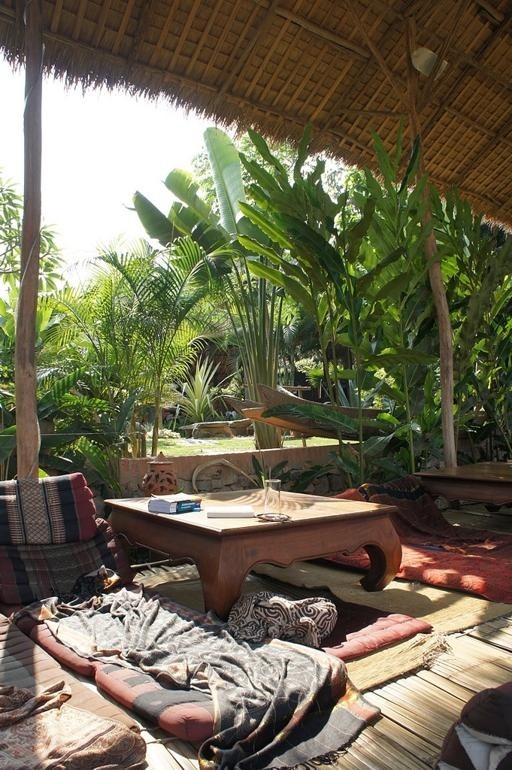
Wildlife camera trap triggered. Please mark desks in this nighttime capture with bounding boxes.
[103,487,403,621]
[412,461,512,508]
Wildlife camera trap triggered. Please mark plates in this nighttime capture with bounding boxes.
[256,513,290,522]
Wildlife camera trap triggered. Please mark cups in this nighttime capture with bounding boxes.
[264,479,281,519]
